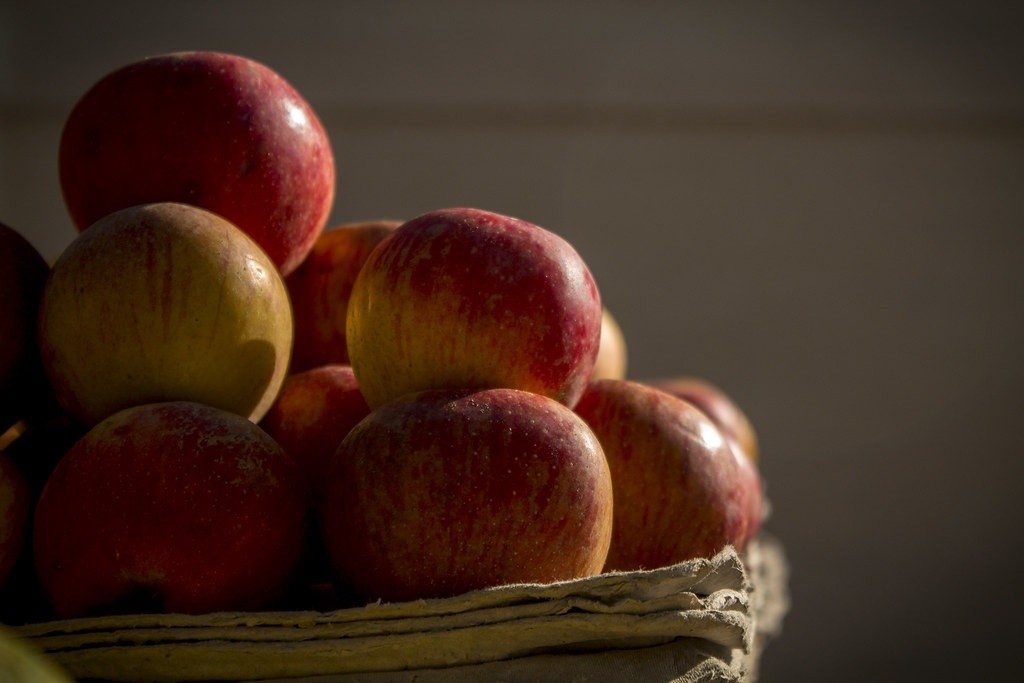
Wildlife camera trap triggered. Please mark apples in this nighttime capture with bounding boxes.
[0,203,766,619]
[57,50,337,277]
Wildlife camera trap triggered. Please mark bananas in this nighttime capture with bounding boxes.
[0,624,74,683]
